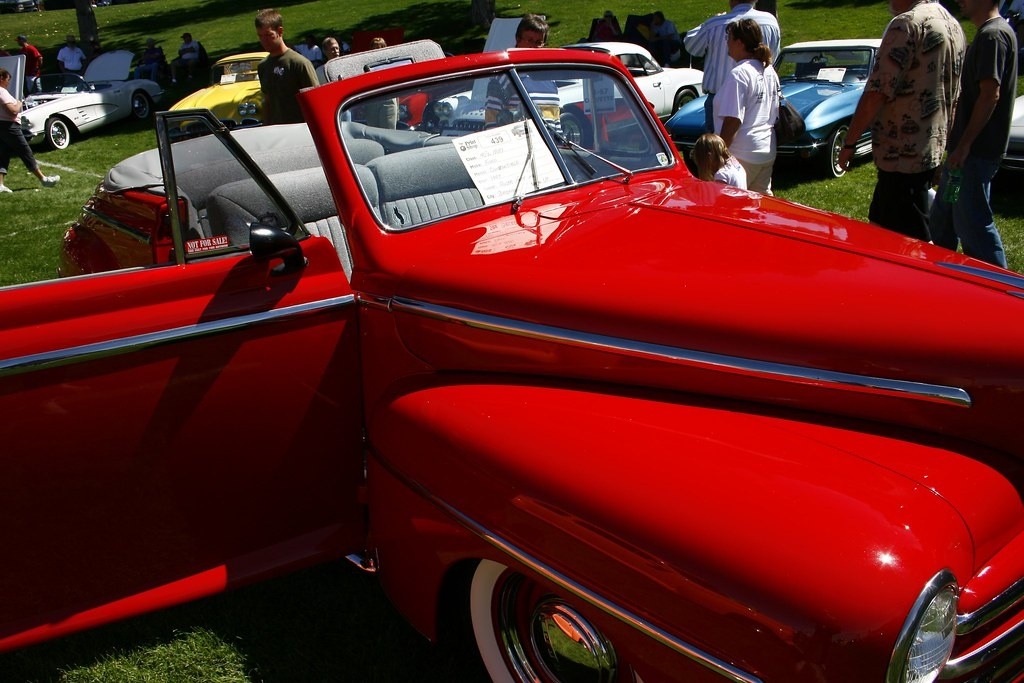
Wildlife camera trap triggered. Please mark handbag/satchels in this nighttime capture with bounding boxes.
[778,96,806,143]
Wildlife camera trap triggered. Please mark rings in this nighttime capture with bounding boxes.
[953,162,958,166]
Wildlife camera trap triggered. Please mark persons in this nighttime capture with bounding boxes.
[713,19,781,198]
[839,0,967,243]
[88,41,101,62]
[647,11,681,67]
[693,134,747,190]
[293,34,324,61]
[485,14,561,144]
[929,0,1018,269]
[169,33,200,86]
[683,0,781,132]
[134,38,165,82]
[56,35,86,84]
[255,8,321,125]
[90,0,112,6]
[15,35,43,95]
[314,36,352,122]
[591,11,620,42]
[33,0,41,11]
[365,38,400,129]
[0,68,60,193]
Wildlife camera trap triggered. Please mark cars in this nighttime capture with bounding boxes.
[396,41,704,151]
[0,50,164,151]
[168,52,271,140]
[996,95,1024,196]
[0,48,1024,683]
[661,39,882,179]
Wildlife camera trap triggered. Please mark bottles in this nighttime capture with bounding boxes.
[943,169,964,203]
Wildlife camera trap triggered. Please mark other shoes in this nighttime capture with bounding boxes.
[0,185,13,194]
[43,175,60,187]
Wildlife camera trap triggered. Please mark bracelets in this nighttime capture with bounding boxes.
[843,145,855,149]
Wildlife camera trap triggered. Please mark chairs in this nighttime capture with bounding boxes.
[205,162,384,283]
[367,144,484,231]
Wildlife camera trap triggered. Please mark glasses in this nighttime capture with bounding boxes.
[725,34,738,41]
[519,37,544,48]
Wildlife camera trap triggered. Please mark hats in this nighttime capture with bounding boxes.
[13,35,28,43]
[181,33,192,38]
[64,35,76,42]
[147,38,157,44]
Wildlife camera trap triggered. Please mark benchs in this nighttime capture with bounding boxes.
[105,122,440,237]
